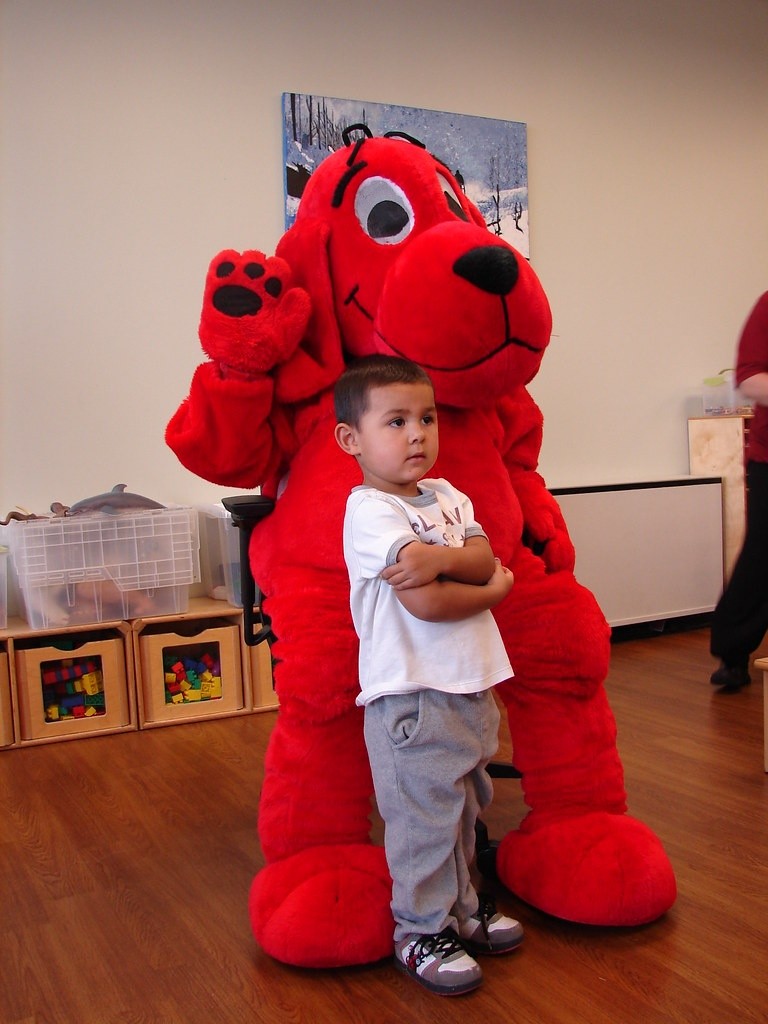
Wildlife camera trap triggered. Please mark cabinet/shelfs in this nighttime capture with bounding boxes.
[0,595,281,752]
[686,414,753,590]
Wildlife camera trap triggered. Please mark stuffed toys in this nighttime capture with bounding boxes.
[164,124,679,969]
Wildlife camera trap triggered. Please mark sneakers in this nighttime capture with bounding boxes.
[456,903,526,957]
[394,925,484,994]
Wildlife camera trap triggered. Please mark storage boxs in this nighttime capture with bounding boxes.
[0,651,14,748]
[15,638,129,743]
[0,545,8,631]
[251,620,282,708]
[139,623,244,723]
[10,506,202,629]
[197,506,227,601]
[215,503,260,609]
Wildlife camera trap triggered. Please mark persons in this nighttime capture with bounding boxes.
[332,356,528,999]
[707,289,768,687]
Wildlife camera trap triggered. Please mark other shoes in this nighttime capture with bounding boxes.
[710,658,752,688]
